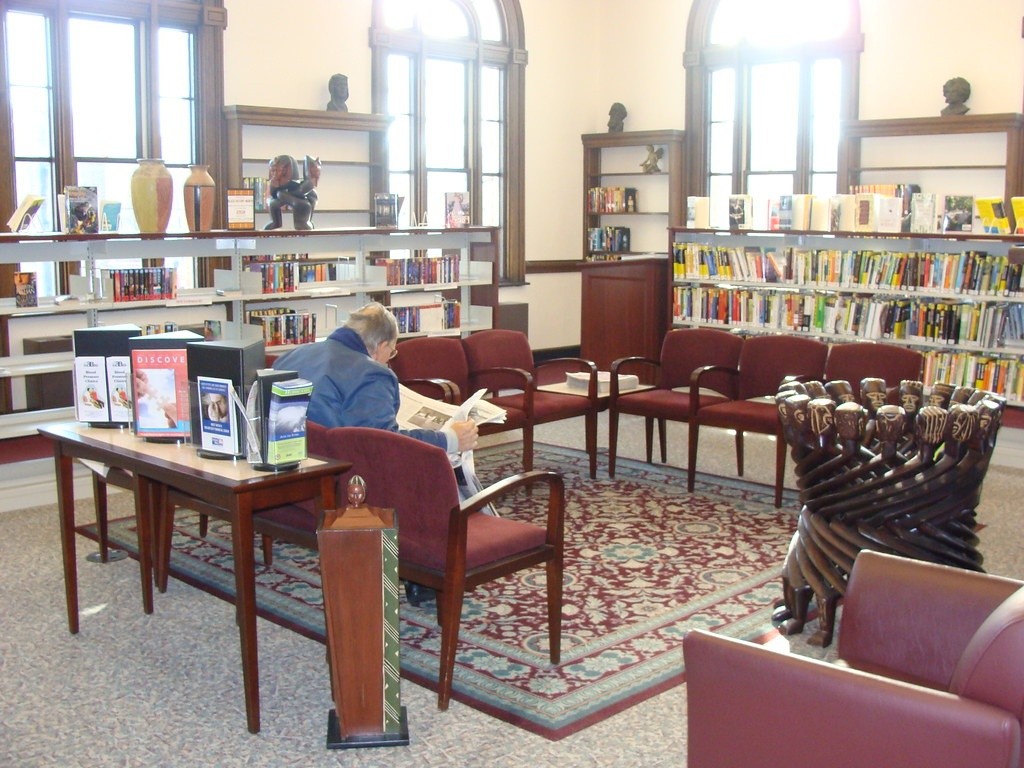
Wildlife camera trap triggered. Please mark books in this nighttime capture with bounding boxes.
[673,184,1024,403]
[375,193,405,230]
[58,186,98,234]
[244,307,316,345]
[101,267,178,302]
[141,319,221,341]
[14,272,38,308]
[385,302,460,333]
[99,199,121,231]
[586,227,630,261]
[73,348,312,465]
[7,194,45,232]
[375,257,459,286]
[586,185,636,214]
[242,254,336,293]
[243,177,304,211]
[445,191,473,229]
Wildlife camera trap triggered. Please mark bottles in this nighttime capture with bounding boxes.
[131,158,173,233]
[183,165,215,232]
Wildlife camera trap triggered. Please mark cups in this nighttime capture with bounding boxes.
[373,193,397,227]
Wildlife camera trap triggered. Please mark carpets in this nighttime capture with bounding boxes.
[70,439,844,745]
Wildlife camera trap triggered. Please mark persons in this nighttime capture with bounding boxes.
[271,302,478,452]
[208,393,228,422]
[326,73,348,112]
[940,77,970,115]
[640,144,661,172]
[136,369,177,428]
[14,272,34,294]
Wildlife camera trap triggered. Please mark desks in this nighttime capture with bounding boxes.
[537,382,657,461]
[36,418,352,734]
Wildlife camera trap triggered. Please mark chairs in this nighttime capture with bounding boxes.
[266,354,453,405]
[681,550,1024,768]
[323,425,570,713]
[250,419,333,570]
[687,338,830,510]
[826,342,926,389]
[462,329,599,495]
[391,337,535,503]
[607,327,744,478]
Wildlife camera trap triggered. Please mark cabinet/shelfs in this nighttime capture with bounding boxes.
[582,128,690,389]
[0,226,500,515]
[225,104,396,258]
[667,228,1024,431]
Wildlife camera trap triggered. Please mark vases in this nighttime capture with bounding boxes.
[184,164,218,233]
[130,158,173,234]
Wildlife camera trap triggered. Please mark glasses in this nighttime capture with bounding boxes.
[387,346,397,359]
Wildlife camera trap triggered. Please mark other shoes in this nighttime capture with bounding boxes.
[405,582,437,607]
